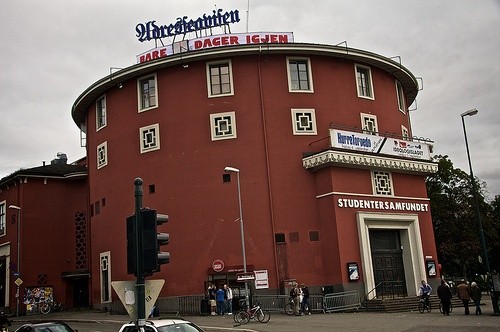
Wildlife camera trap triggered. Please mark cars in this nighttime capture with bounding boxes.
[15,321,79,332]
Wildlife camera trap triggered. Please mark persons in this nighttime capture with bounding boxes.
[437,280,451,316]
[456,278,470,315]
[216,286,225,316]
[418,279,433,310]
[289,282,298,313]
[299,283,312,316]
[466,280,471,301]
[223,284,234,316]
[468,281,482,316]
[208,284,217,315]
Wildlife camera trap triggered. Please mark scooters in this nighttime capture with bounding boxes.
[0,322,9,332]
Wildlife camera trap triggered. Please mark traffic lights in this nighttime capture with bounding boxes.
[140,207,172,279]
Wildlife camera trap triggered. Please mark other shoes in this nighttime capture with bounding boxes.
[301,313,304,315]
[211,311,216,316]
[228,312,232,316]
[308,312,311,316]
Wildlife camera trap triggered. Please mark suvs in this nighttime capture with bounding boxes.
[118,317,205,332]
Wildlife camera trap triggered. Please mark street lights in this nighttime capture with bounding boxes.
[224,167,251,316]
[9,205,21,317]
[460,108,500,316]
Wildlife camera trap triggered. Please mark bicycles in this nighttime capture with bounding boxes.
[41,299,64,315]
[417,295,431,313]
[232,300,271,325]
[284,297,311,317]
